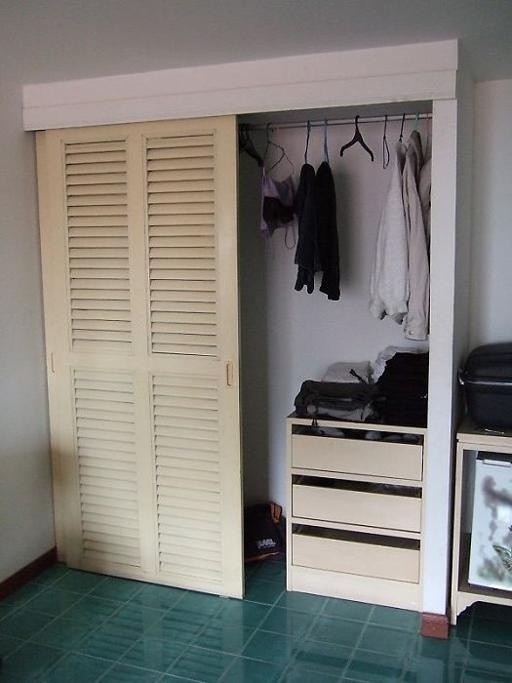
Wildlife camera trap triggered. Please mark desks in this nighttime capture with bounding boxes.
[449,418,512,626]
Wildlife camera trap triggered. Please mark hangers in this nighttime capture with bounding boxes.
[260,112,428,174]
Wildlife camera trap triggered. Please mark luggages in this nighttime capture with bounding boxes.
[459,340,512,430]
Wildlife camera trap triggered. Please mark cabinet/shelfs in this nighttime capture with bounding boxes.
[32,115,268,601]
[286,410,427,613]
